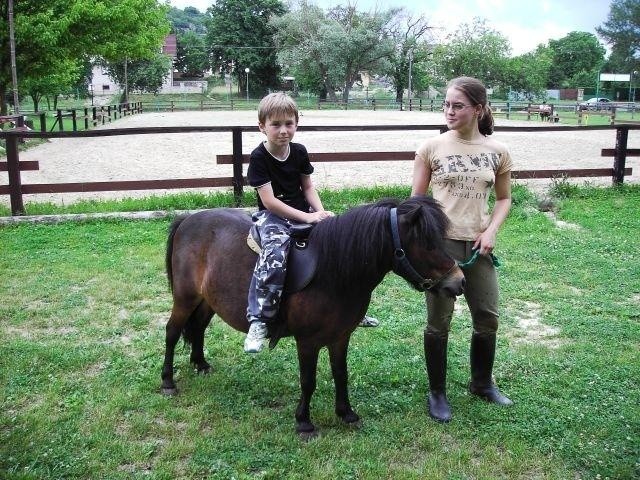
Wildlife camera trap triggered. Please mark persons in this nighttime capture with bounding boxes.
[539,102,553,122]
[411,77,515,423]
[244,93,334,353]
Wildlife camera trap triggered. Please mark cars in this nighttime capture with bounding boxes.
[578,98,614,110]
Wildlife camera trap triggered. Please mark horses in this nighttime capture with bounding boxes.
[160,193,468,441]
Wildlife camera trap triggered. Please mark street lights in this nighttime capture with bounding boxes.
[244,68,250,102]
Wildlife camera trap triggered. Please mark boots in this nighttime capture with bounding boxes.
[470,332,513,406]
[424,333,452,422]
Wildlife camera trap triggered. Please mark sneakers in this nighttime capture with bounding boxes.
[244,322,268,353]
[359,316,378,327]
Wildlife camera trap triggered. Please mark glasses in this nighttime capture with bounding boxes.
[444,102,474,111]
